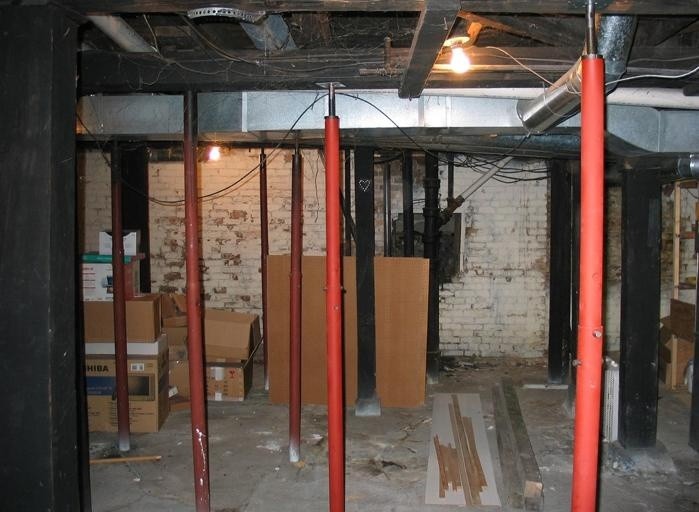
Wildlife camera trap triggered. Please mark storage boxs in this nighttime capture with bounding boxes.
[82,225,261,433]
[658,298,697,392]
